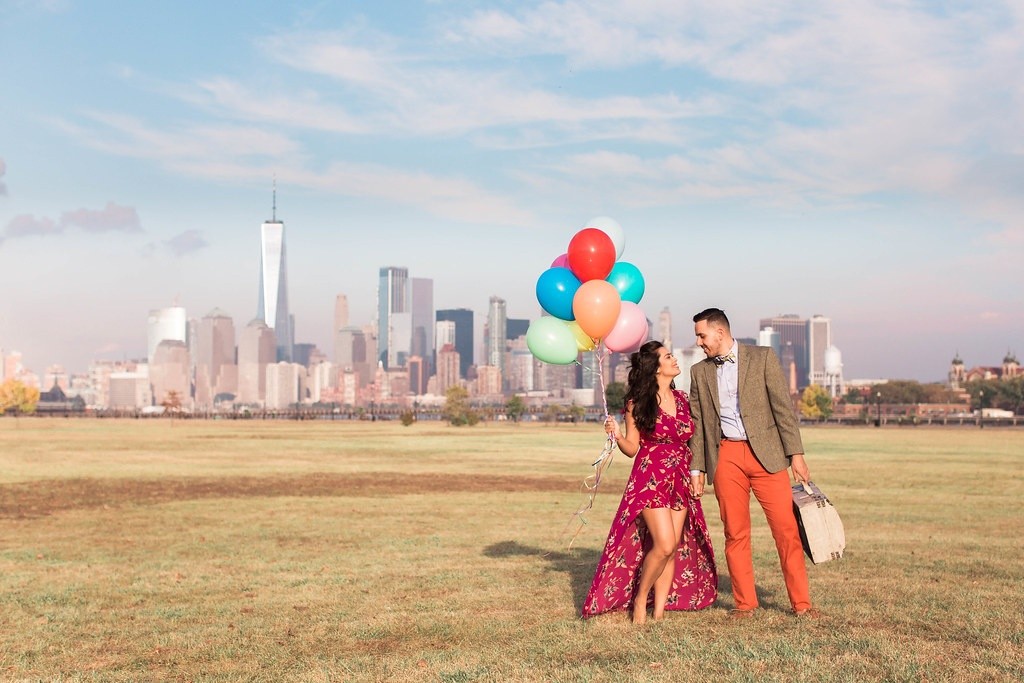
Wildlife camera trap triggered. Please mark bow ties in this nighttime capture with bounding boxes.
[715,351,736,368]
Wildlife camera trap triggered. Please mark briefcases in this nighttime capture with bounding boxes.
[791,479,846,565]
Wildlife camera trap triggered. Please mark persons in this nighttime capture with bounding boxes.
[582,309,814,626]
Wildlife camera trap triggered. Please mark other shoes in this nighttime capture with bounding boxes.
[794,608,831,619]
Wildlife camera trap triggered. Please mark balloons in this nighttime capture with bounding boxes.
[526,217,649,365]
